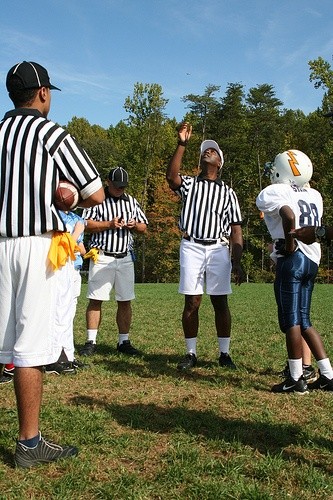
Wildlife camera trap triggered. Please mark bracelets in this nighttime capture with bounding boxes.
[177,141,188,147]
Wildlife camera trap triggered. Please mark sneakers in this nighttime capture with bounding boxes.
[15,431,78,467]
[65,359,91,370]
[0,368,14,384]
[271,374,308,396]
[45,361,77,375]
[306,373,333,391]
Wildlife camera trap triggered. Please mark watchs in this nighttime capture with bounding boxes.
[313,225,331,247]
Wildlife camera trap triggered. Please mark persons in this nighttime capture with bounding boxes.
[80,166,148,358]
[165,120,247,372]
[255,149,333,394]
[0,61,105,468]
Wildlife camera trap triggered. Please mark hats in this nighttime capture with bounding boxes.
[6,61,62,92]
[108,167,130,187]
[201,140,224,171]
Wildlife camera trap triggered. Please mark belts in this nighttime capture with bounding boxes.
[183,234,228,246]
[97,250,132,259]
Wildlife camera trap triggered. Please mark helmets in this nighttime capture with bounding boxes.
[270,150,313,188]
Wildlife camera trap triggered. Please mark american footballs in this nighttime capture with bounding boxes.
[55,181,81,212]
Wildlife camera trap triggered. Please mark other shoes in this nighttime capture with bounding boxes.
[117,340,138,354]
[177,353,197,370]
[79,340,98,357]
[218,351,236,370]
[274,361,290,378]
[303,365,317,381]
